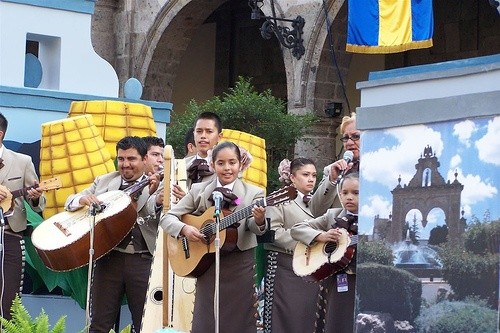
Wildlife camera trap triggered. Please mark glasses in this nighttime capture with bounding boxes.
[340,134,360,142]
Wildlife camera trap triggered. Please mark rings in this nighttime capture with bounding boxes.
[29,196,34,199]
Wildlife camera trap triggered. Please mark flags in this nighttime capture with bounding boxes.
[346,0,434,55]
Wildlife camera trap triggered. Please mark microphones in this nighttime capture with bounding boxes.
[336,150,354,183]
[213,191,223,215]
[90,202,101,212]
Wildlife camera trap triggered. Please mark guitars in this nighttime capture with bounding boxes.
[30,167,165,273]
[293,228,358,284]
[0,176,63,216]
[167,184,299,279]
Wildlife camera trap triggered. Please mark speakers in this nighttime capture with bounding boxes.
[19,294,134,333]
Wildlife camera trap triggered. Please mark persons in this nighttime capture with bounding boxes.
[308,112,360,218]
[64,136,160,333]
[290,173,359,333]
[170,111,224,207]
[0,113,46,333]
[263,157,317,333]
[160,142,268,333]
[183,126,200,159]
[131,136,173,333]
